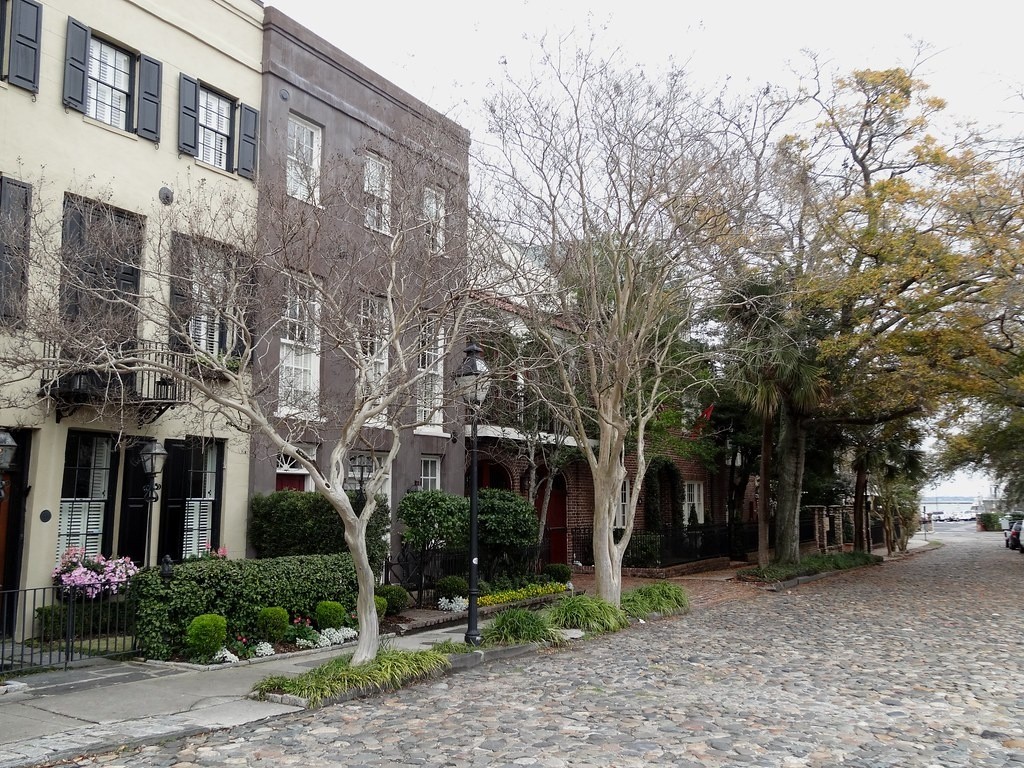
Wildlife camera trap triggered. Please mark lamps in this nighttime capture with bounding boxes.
[70,369,92,404]
[155,375,177,402]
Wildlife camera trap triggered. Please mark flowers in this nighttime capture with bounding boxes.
[50,545,142,604]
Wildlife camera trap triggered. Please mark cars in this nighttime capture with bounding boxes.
[1009,523,1022,549]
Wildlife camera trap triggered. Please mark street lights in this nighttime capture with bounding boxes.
[450,345,493,647]
